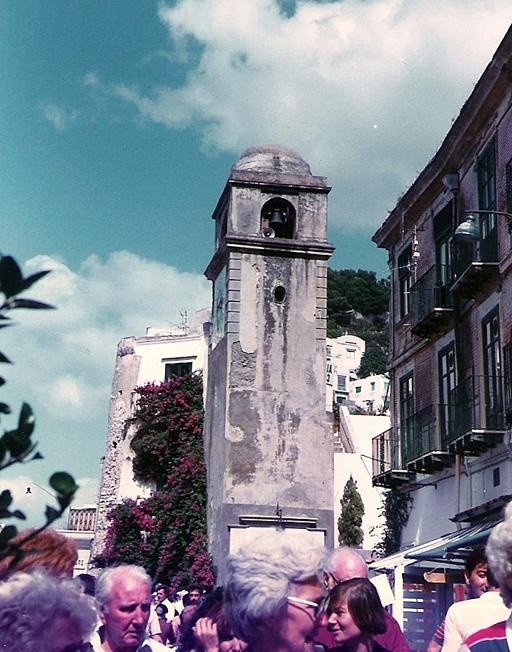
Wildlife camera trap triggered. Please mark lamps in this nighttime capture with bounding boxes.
[451,209,506,244]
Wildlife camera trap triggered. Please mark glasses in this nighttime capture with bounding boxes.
[286,594,332,621]
